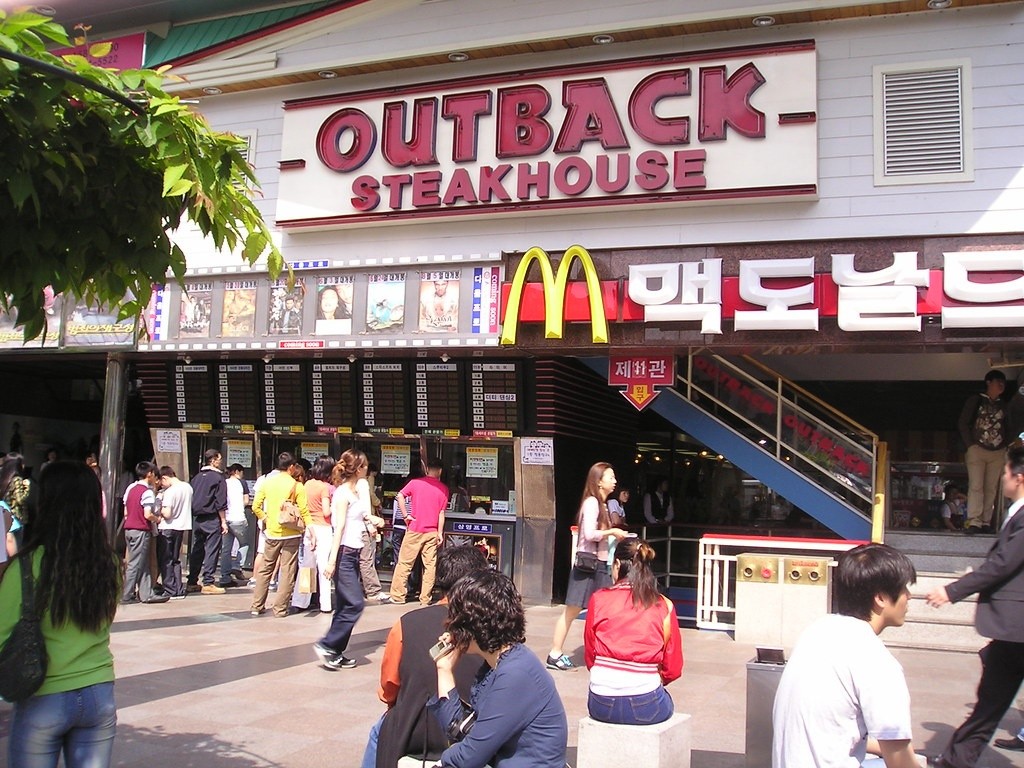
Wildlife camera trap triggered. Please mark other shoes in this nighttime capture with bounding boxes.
[917,752,954,768]
[368,593,389,602]
[963,526,982,534]
[995,736,1024,750]
[125,569,287,617]
[381,598,393,604]
[982,525,993,533]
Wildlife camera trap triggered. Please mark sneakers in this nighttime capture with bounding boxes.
[546,654,576,671]
[312,643,342,670]
[341,657,357,668]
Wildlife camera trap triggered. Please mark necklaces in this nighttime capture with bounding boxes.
[348,480,359,494]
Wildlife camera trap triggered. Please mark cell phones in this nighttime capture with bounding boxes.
[428,640,455,661]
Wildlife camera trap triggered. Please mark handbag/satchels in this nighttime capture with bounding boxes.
[279,481,305,532]
[575,552,598,573]
[0,552,46,702]
[299,549,317,594]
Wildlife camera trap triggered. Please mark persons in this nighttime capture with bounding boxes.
[546,462,628,672]
[383,456,449,606]
[179,280,459,334]
[607,490,629,527]
[583,537,684,726]
[773,541,927,768]
[0,439,335,618]
[0,460,121,768]
[314,446,391,672]
[749,490,786,520]
[918,445,1024,768]
[643,477,674,553]
[959,369,1011,534]
[938,483,969,532]
[361,544,490,768]
[398,567,568,768]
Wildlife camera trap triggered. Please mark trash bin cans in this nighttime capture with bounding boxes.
[746,641,788,768]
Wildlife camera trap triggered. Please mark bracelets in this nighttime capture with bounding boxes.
[402,512,409,520]
